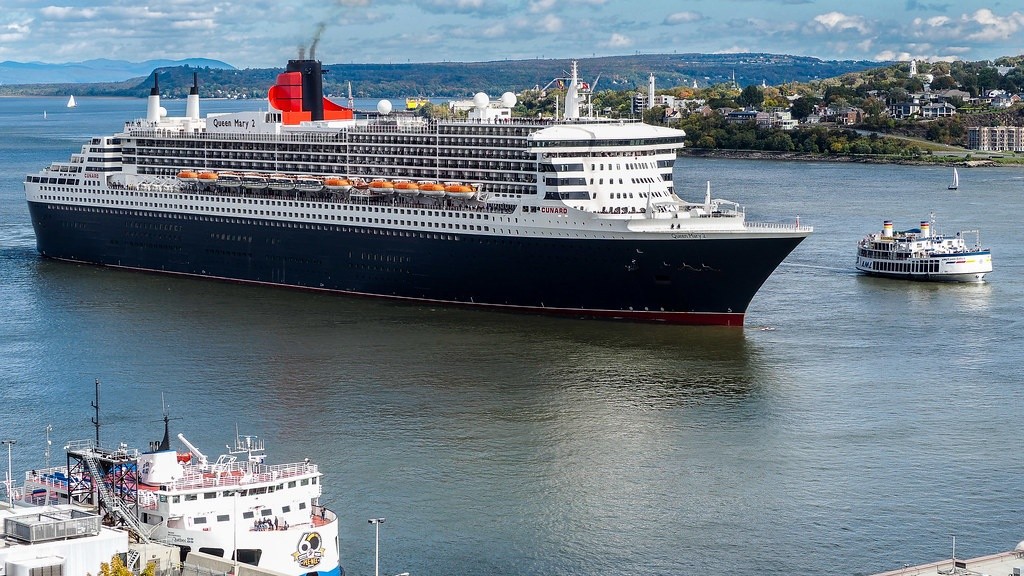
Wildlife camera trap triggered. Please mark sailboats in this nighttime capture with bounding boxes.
[947,167,959,190]
[67,95,77,108]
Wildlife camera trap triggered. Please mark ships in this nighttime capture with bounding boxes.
[20,58,815,328]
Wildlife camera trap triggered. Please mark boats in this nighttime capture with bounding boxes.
[354,181,369,190]
[403,97,430,110]
[268,175,295,191]
[4,381,342,576]
[369,178,393,193]
[323,177,351,192]
[216,172,242,186]
[197,172,218,183]
[855,220,994,284]
[418,183,445,198]
[177,171,197,183]
[295,177,324,191]
[242,174,268,190]
[445,184,476,200]
[393,180,418,196]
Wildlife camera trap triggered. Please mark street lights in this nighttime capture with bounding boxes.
[3,441,18,508]
[369,517,384,575]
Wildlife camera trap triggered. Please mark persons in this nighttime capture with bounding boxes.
[321,507,325,520]
[32,469,37,480]
[254,518,274,531]
[274,516,279,530]
[283,521,289,530]
